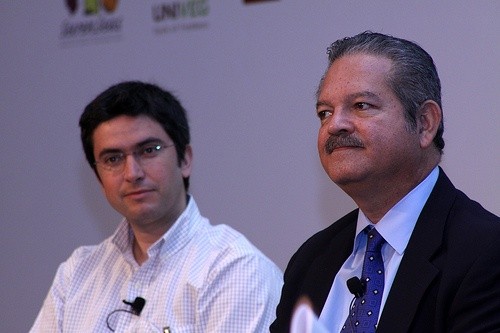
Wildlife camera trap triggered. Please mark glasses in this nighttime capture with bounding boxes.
[94,140,174,167]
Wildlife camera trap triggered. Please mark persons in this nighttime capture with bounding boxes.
[27,80,284,333]
[269,31,499,332]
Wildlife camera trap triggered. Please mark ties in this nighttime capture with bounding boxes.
[339,226,386,333]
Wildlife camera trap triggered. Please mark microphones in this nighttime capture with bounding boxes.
[131,297,145,316]
[347,276,363,298]
